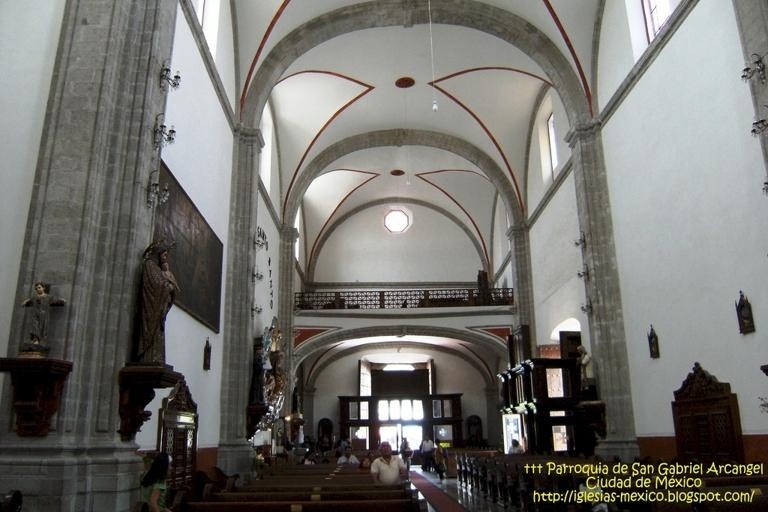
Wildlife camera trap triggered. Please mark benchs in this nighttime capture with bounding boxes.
[172,442,768,512]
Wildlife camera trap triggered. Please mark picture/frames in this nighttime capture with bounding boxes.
[150,156,224,335]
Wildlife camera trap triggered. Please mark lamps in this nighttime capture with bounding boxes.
[249,235,264,318]
[426,1,440,115]
[573,230,588,252]
[749,110,766,139]
[578,297,593,318]
[576,263,590,284]
[144,61,180,209]
[738,52,767,89]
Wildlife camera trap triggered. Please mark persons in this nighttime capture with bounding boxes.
[20,279,67,345]
[136,241,180,365]
[282,428,445,486]
[578,474,608,511]
[140,454,173,512]
[508,439,524,454]
[576,345,595,391]
